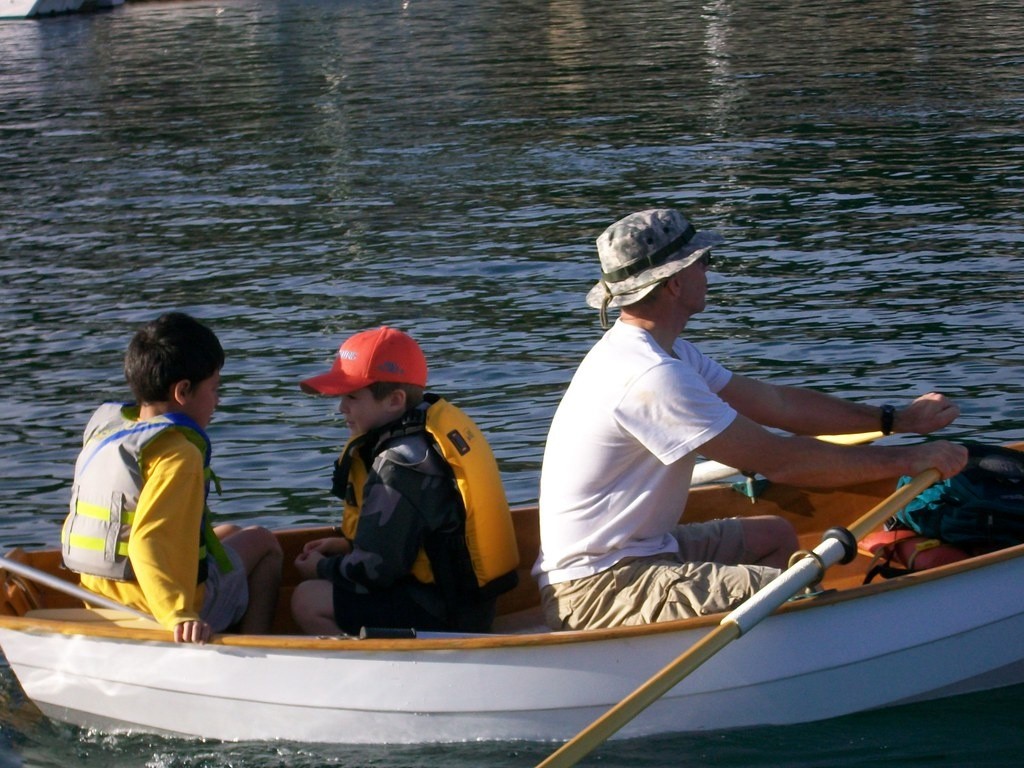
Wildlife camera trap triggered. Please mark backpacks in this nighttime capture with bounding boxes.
[886,445,1024,555]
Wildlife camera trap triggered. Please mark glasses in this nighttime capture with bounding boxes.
[660,250,712,287]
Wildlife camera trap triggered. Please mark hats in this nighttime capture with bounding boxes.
[299,325,427,394]
[586,209,719,308]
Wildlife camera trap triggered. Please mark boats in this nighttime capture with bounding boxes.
[0,438,1024,742]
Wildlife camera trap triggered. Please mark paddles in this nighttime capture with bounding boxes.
[534,463,942,767]
[690,428,895,485]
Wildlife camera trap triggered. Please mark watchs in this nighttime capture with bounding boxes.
[880,405,895,436]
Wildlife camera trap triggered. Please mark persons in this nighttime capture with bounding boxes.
[290,325,521,635]
[62,312,283,646]
[532,209,968,631]
